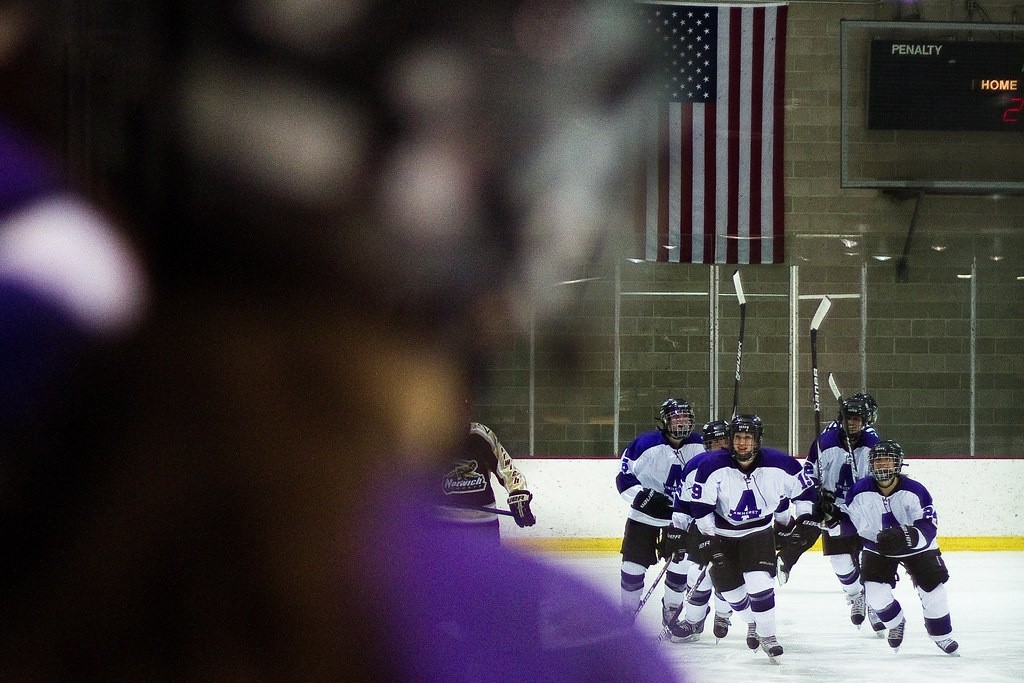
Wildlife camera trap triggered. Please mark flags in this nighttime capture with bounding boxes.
[608,1,785,264]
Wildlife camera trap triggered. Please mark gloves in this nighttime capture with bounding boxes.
[632,488,673,518]
[663,527,687,564]
[877,525,919,555]
[505,489,538,527]
[774,515,797,538]
[694,535,724,565]
[799,511,821,552]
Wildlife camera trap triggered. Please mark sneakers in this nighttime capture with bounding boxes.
[866,608,886,639]
[850,594,867,629]
[659,597,684,634]
[746,622,761,654]
[669,617,704,643]
[886,618,906,651]
[760,635,784,664]
[935,639,961,657]
[713,613,730,644]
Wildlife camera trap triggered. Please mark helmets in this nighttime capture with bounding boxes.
[868,439,902,482]
[836,392,877,435]
[729,413,763,459]
[702,420,730,451]
[660,396,696,439]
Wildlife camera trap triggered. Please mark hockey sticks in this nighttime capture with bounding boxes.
[825,371,859,482]
[808,294,833,488]
[654,561,714,646]
[630,551,676,623]
[729,268,747,422]
[437,498,538,521]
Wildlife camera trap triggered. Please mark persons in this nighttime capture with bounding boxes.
[1,0,961,682]
